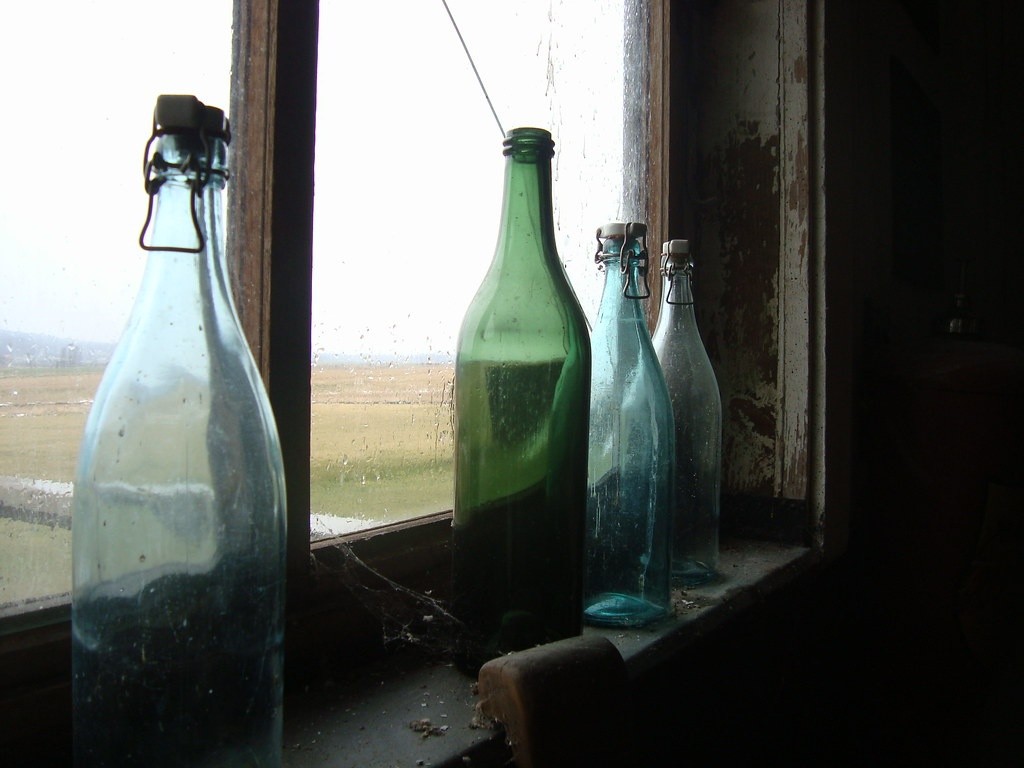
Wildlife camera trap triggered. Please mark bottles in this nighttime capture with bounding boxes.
[71,93,290,768]
[586,223,673,627]
[650,240,723,589]
[452,124,593,674]
